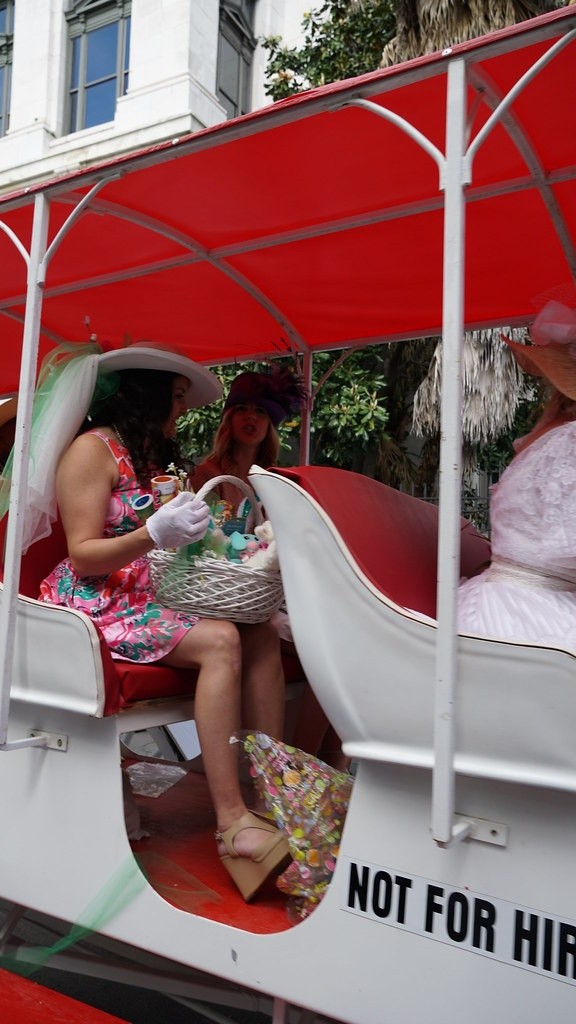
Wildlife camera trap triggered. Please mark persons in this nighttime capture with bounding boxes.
[1,341,349,901]
[457,300,575,652]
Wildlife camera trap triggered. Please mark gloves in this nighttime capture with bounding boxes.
[146,492,210,549]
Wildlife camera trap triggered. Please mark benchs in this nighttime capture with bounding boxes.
[248,466,576,784]
[0,499,194,720]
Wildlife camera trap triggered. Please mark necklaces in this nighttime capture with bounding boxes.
[113,424,127,447]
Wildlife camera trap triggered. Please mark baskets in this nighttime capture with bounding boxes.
[147,475,285,624]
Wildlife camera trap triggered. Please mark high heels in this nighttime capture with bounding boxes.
[213,812,291,903]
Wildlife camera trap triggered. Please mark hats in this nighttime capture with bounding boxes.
[222,361,306,426]
[0,341,221,556]
[500,300,576,402]
[0,397,17,426]
